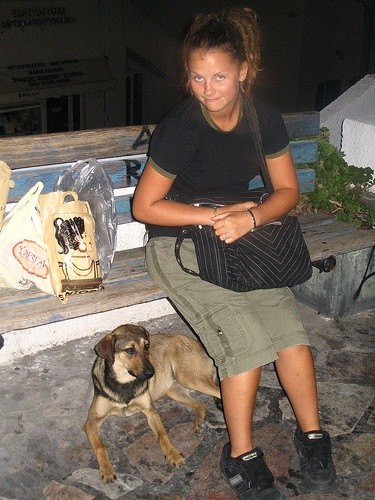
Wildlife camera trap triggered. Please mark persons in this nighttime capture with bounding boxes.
[131,6,338,500]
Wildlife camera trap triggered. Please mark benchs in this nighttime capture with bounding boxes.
[0,111,375,334]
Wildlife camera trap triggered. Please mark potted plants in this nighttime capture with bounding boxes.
[285,125,375,319]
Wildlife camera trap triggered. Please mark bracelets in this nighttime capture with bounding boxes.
[247,209,256,229]
[213,206,218,216]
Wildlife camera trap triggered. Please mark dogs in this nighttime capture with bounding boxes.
[83,324,222,485]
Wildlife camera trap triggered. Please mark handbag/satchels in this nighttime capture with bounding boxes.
[0,158,118,305]
[175,190,313,293]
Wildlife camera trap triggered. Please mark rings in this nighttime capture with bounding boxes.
[225,233,229,239]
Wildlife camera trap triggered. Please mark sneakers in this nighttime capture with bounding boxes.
[219,442,281,500]
[293,424,336,493]
